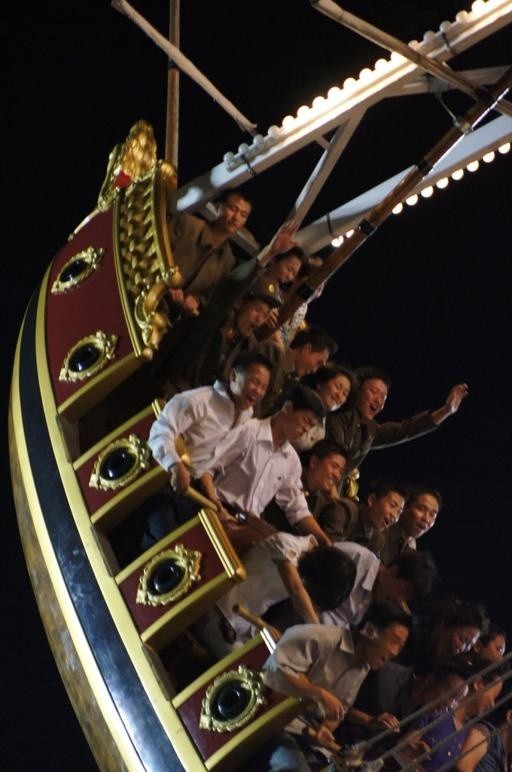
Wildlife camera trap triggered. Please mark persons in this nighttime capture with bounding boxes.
[95,189,510,771]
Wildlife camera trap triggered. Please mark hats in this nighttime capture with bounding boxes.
[246,274,282,308]
